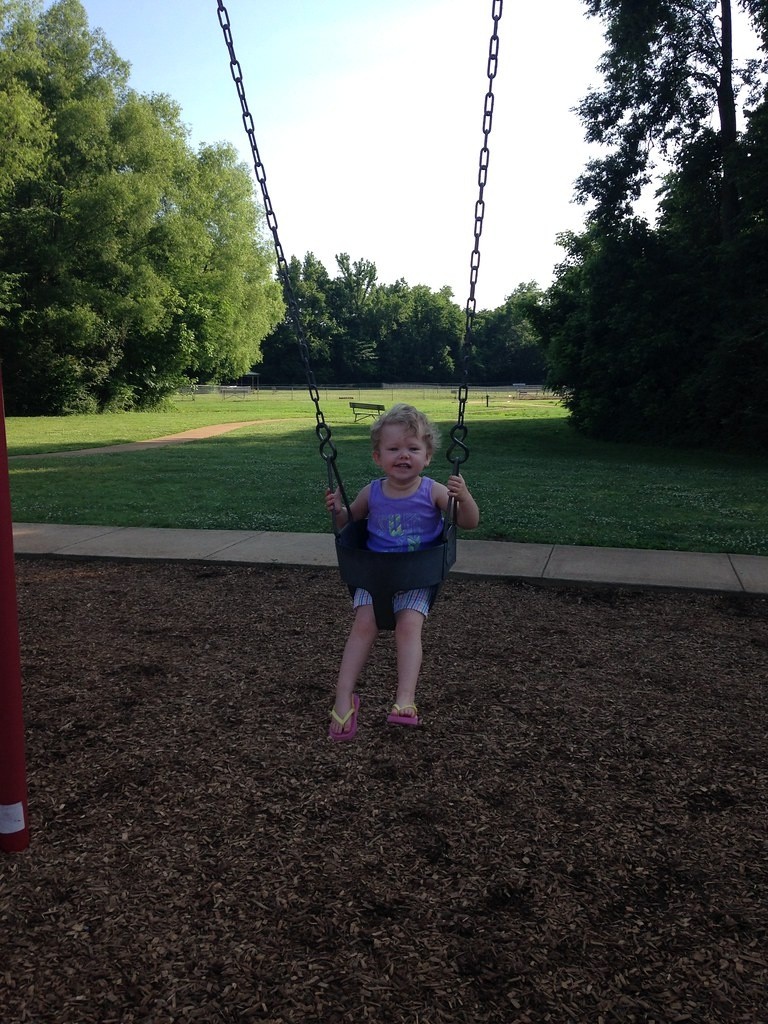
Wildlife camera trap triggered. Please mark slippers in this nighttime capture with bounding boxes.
[386,704,419,726]
[329,694,360,739]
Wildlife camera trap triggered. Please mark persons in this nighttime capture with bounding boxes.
[326,404,480,741]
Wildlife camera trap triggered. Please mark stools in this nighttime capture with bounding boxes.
[349,402,386,423]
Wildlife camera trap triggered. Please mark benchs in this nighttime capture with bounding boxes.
[221,385,251,401]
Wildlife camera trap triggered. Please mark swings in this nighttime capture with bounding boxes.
[215,0,504,632]
